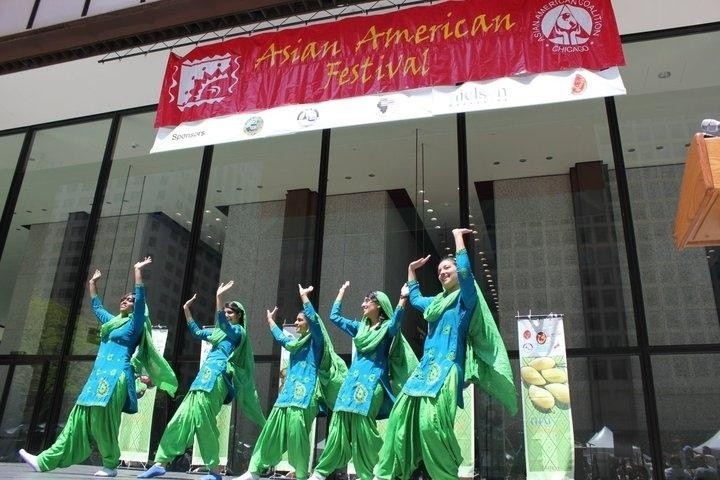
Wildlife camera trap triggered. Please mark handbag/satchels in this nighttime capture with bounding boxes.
[126,358,152,401]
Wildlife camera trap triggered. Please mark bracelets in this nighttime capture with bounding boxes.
[399,295,408,299]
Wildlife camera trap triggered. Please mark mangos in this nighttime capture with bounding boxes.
[527,358,555,371]
[545,383,569,403]
[529,385,555,408]
[541,368,567,384]
[520,367,547,386]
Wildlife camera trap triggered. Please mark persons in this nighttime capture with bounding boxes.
[305,279,411,480]
[136,280,248,480]
[582,443,720,480]
[17,255,154,478]
[231,283,334,480]
[371,227,480,480]
